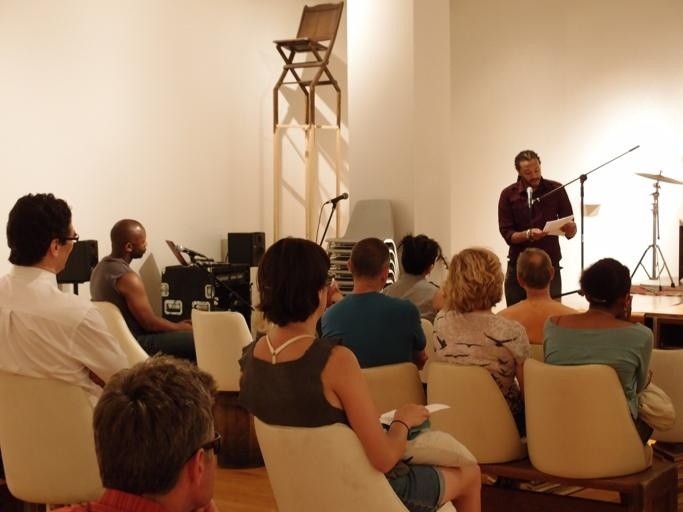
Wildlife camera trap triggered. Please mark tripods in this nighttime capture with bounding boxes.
[631,191,677,292]
[535,145,640,299]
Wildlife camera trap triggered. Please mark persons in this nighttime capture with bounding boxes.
[499,150,576,305]
[90,219,196,363]
[322,238,427,367]
[543,257,654,445]
[492,249,581,345]
[0,193,131,411]
[425,248,528,435]
[382,233,444,326]
[236,236,481,510]
[47,351,219,512]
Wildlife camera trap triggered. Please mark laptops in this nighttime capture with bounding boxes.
[166,240,213,266]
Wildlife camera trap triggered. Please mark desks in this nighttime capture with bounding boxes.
[553,284,683,348]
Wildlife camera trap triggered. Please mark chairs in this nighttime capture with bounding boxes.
[524,362,651,478]
[253,416,456,512]
[274,3,343,90]
[424,365,520,462]
[1,371,111,505]
[91,301,150,372]
[192,310,264,471]
[361,364,423,422]
[325,199,399,295]
[645,349,682,464]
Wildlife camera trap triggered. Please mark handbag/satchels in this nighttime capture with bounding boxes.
[640,382,676,431]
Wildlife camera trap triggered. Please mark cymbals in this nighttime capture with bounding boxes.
[635,171,682,186]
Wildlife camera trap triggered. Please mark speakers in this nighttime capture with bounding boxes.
[228,232,265,265]
[57,239,99,284]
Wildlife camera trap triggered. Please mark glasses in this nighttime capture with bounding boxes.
[188,430,221,465]
[62,233,79,244]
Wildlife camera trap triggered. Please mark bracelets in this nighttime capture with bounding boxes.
[389,419,410,430]
[527,229,532,242]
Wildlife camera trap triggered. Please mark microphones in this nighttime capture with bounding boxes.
[175,243,209,260]
[525,186,534,207]
[321,192,348,204]
